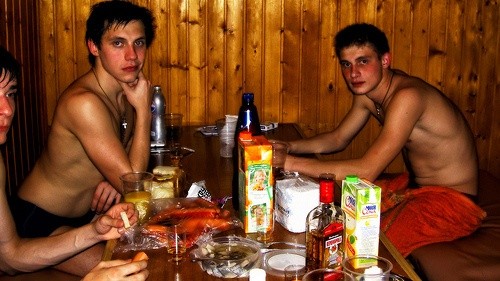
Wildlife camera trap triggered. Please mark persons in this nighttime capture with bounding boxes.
[268,22,487,258]
[0,45,150,281]
[7,0,158,276]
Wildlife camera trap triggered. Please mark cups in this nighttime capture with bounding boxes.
[119,172,154,220]
[302,268,354,281]
[341,254,393,281]
[167,232,187,266]
[270,141,288,190]
[215,117,237,158]
[164,113,184,150]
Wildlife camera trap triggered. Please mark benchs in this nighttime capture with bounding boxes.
[379,172,500,281]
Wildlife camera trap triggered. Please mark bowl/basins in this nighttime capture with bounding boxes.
[188,236,262,278]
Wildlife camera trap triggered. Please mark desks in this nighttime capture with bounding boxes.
[102,123,422,281]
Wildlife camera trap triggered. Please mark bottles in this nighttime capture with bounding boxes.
[149,85,166,147]
[234,93,261,164]
[169,146,184,168]
[305,173,347,281]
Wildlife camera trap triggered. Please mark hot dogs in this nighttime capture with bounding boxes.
[143,207,235,234]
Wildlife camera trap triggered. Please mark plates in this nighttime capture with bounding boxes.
[200,125,218,135]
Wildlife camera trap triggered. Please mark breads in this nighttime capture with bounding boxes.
[132,251,149,262]
[120,212,130,228]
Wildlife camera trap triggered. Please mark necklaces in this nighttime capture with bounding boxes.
[91,68,128,129]
[374,73,395,115]
